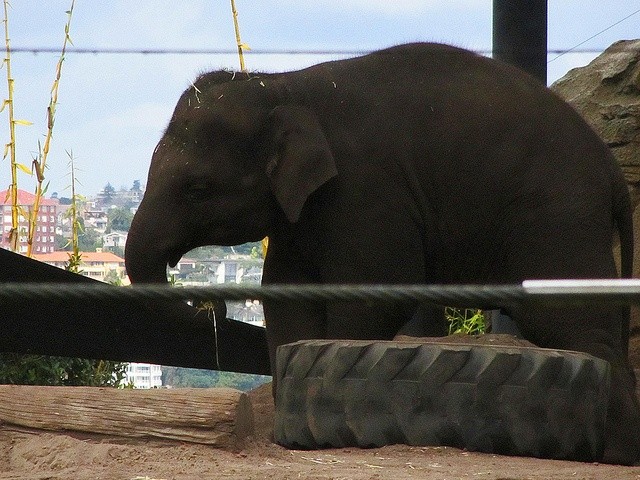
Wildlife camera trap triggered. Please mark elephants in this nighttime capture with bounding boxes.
[123,39,639,466]
